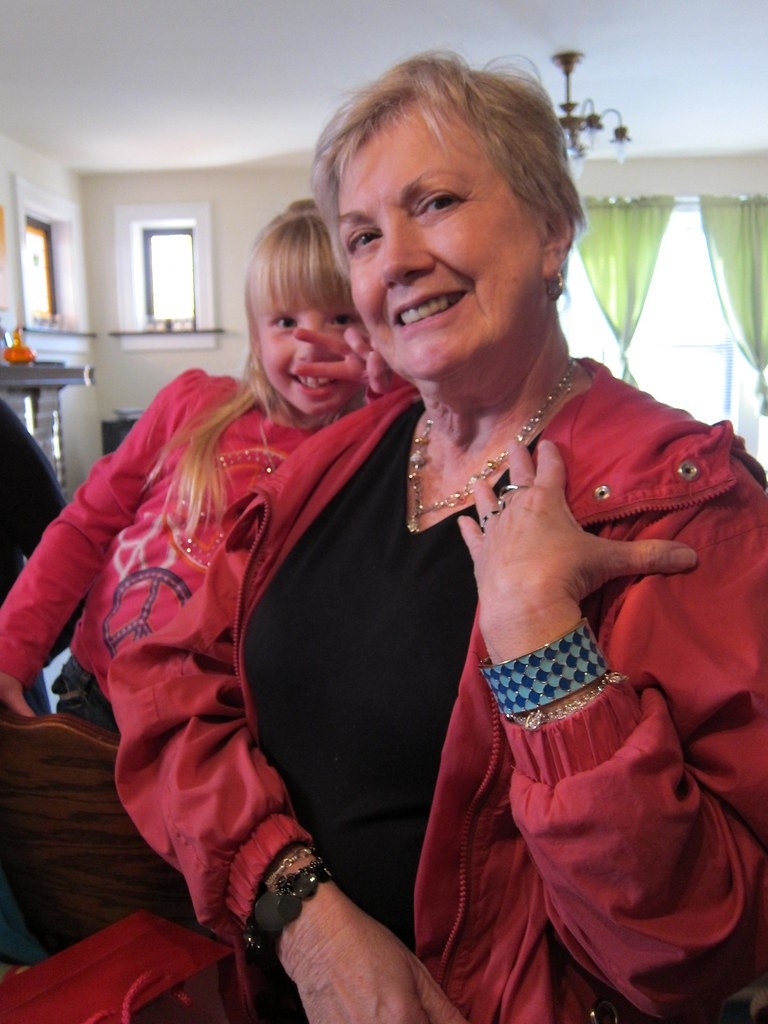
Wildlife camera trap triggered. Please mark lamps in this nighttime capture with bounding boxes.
[548,52,631,165]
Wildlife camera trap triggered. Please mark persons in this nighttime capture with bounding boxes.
[107,48,768,1024]
[0,199,411,732]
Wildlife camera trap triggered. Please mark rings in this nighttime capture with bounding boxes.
[498,484,531,513]
[480,510,499,528]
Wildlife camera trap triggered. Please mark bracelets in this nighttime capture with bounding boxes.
[477,618,630,731]
[242,845,332,971]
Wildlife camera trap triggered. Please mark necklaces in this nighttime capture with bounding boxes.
[408,356,582,534]
[260,415,277,472]
